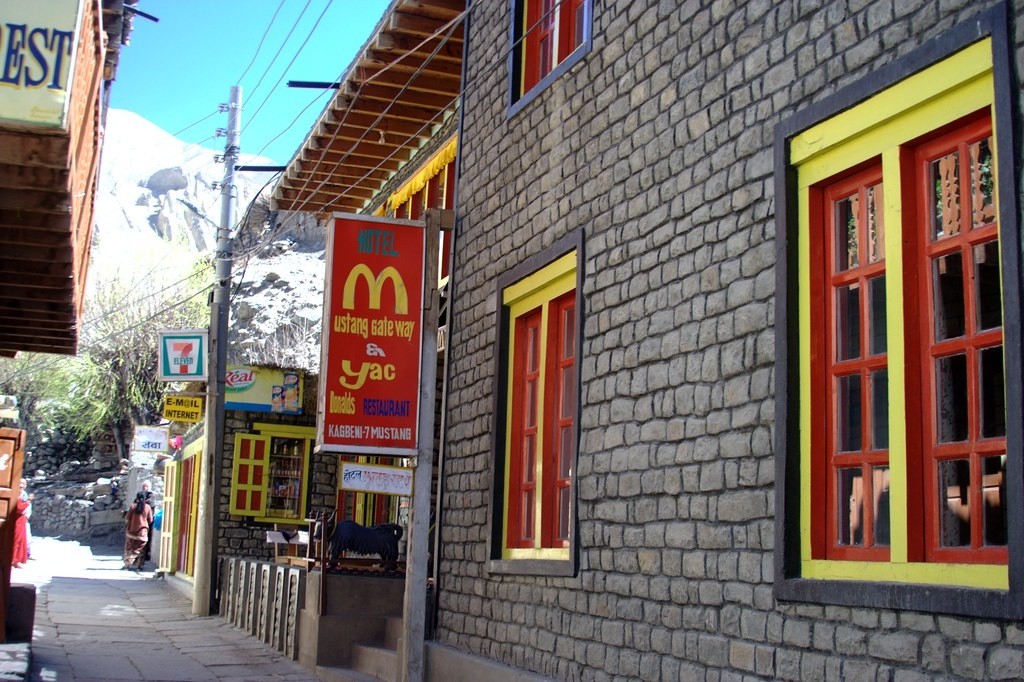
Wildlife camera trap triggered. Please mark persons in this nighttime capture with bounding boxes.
[20,479,34,561]
[121,484,156,572]
[11,484,35,569]
